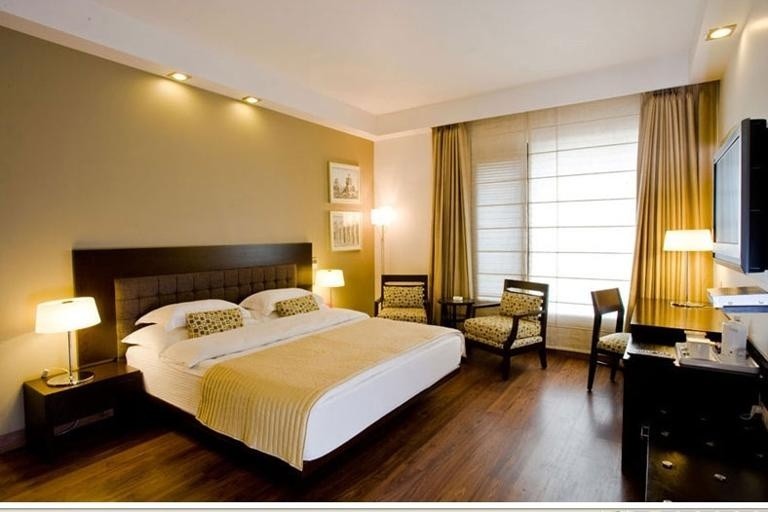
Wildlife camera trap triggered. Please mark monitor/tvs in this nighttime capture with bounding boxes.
[711,117,768,274]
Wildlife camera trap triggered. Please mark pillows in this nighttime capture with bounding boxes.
[382,286,425,307]
[500,290,541,322]
[121,289,324,352]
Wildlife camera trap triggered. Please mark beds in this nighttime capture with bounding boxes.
[72,242,466,492]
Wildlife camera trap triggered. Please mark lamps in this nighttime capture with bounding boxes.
[33,295,102,385]
[663,228,713,308]
[316,269,344,306]
[371,208,396,274]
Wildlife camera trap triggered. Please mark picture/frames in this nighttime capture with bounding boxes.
[330,211,364,252]
[328,161,361,204]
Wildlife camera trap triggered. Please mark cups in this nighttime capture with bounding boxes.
[721,320,748,365]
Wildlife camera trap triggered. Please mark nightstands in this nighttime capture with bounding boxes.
[23,362,141,461]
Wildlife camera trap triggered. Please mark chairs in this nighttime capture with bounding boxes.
[375,275,434,325]
[463,279,548,381]
[588,289,632,392]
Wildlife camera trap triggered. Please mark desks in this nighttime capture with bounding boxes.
[621,340,768,502]
[439,295,474,332]
[630,302,729,349]
